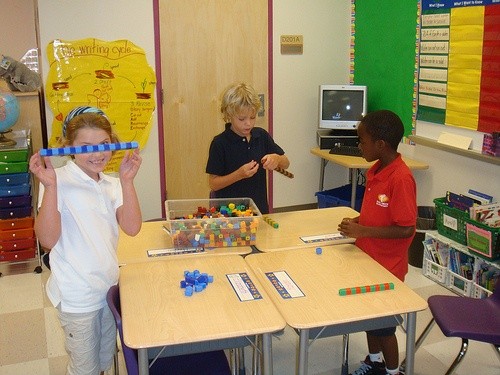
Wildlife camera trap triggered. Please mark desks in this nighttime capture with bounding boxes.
[311,146,431,210]
[250,203,360,254]
[245,243,430,375]
[118,253,287,375]
[116,216,255,267]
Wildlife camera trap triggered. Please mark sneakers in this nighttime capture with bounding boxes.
[349,354,405,375]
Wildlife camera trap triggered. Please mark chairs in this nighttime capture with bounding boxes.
[395,275,500,375]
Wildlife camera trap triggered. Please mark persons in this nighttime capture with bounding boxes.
[29,106,144,375]
[337,110,417,375]
[206,80,290,217]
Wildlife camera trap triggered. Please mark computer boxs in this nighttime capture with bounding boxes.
[316,129,360,150]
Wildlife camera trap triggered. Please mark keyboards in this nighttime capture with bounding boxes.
[329,146,363,157]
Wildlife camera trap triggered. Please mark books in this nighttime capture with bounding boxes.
[474,262,500,299]
[448,247,479,291]
[446,188,493,212]
[469,201,500,227]
[422,238,449,270]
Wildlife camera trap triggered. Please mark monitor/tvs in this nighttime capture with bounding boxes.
[319,85,366,136]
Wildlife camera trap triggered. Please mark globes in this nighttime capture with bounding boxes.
[1,93,20,149]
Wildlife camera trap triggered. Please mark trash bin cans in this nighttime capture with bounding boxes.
[409,205,437,270]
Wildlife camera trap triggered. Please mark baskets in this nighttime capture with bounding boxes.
[424,258,494,298]
[432,197,471,246]
[416,205,437,230]
[463,214,500,263]
